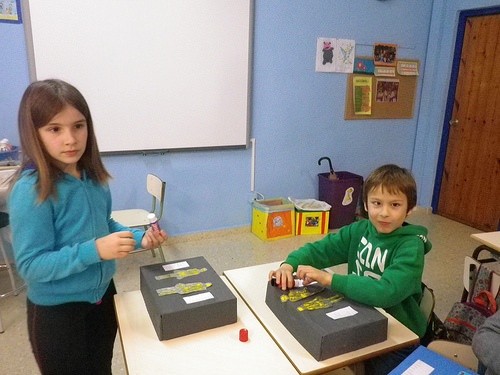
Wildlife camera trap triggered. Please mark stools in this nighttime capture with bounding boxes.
[0,212,17,333]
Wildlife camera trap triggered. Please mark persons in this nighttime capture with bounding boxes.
[6,78,169,375]
[472,311,500,375]
[267,163,432,375]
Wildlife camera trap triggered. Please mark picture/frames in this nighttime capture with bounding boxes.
[0,0,21,23]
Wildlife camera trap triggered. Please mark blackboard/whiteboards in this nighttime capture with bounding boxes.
[21,0,255,157]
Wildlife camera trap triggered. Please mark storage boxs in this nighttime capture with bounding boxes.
[265,270,389,362]
[252,171,365,242]
[387,345,479,375]
[140,256,238,341]
[0,146,19,163]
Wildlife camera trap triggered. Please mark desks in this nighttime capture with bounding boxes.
[462,230,500,303]
[114,260,420,375]
[0,163,20,243]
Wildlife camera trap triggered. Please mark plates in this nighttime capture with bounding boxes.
[286,197,331,212]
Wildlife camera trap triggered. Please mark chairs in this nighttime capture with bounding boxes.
[111,174,167,266]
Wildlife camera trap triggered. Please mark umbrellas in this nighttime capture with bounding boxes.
[318,156,338,180]
